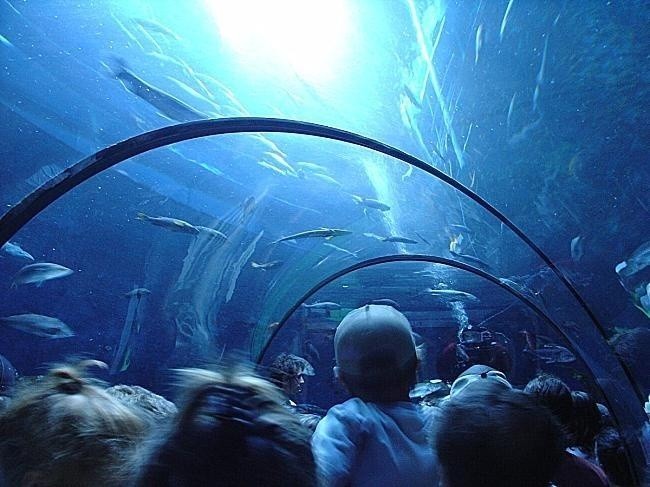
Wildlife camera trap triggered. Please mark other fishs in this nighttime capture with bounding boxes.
[0,0,650,421]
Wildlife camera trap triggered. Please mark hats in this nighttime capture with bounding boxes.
[333,304,416,381]
[450,364,512,398]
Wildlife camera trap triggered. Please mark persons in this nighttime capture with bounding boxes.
[411,364,650,487]
[310,305,442,487]
[1,359,322,487]
[437,340,511,384]
[270,351,305,403]
